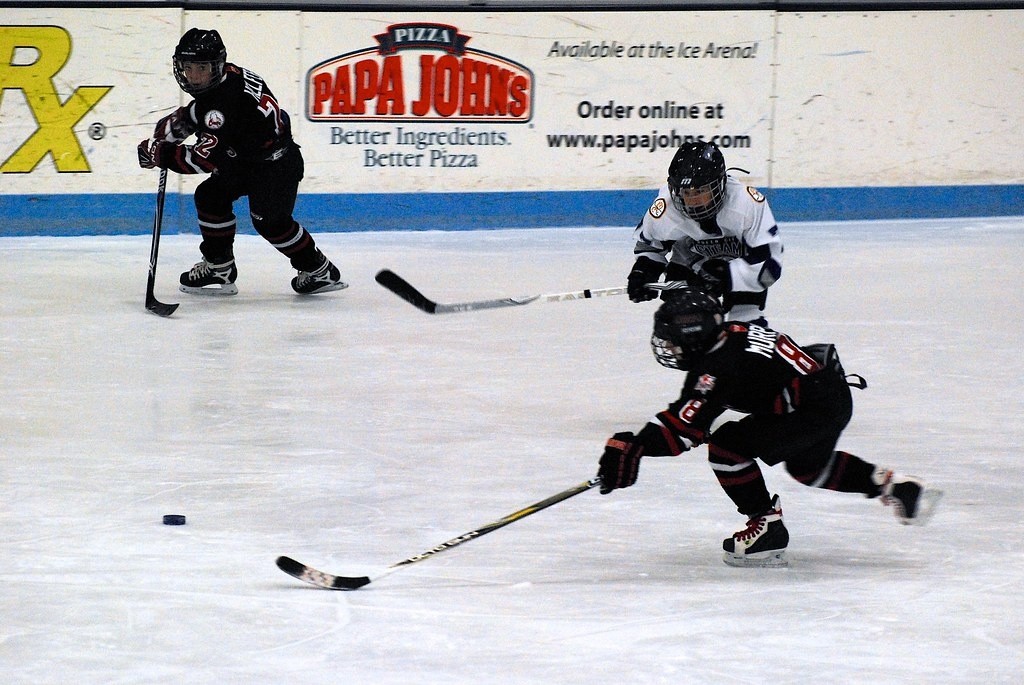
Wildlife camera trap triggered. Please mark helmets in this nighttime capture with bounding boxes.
[650,288,724,367]
[172,28,227,62]
[668,141,727,191]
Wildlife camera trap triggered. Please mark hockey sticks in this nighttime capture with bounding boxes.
[374,269,689,316]
[145,168,180,316]
[274,476,603,592]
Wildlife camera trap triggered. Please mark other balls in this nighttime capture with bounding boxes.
[163,514,186,526]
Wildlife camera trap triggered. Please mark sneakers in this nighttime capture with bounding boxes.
[723,494,790,567]
[291,260,349,295]
[868,464,942,526]
[179,257,237,294]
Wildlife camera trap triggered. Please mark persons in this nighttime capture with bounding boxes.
[627,141,783,326]
[138,28,349,295]
[600,292,940,567]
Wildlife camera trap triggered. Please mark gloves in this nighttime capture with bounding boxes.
[138,138,156,169]
[154,108,166,144]
[598,431,641,494]
[628,257,664,302]
